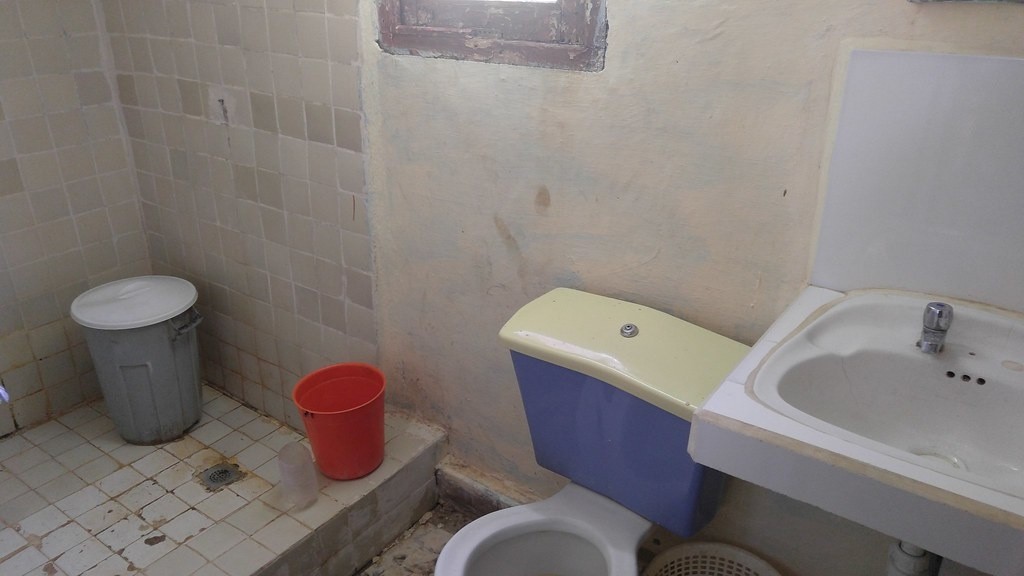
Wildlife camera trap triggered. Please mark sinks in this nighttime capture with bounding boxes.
[686,284,1024,534]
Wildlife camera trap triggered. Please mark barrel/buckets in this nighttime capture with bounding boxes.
[293,363,387,480]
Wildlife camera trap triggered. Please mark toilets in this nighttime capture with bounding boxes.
[433,284,753,576]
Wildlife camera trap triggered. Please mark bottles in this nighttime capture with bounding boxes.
[278,440,319,510]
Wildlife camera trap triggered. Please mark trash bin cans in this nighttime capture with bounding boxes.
[69,274,205,447]
[642,542,781,576]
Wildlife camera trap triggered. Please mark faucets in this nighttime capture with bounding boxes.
[916,300,954,361]
